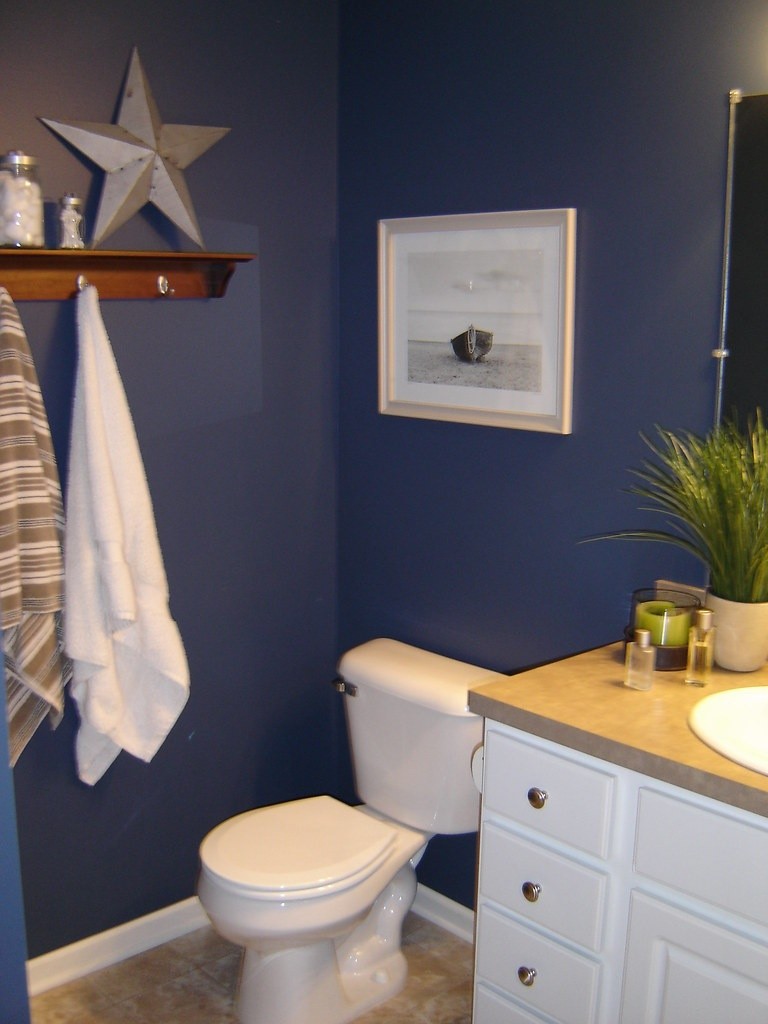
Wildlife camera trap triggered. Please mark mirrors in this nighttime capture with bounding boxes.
[713,89,768,450]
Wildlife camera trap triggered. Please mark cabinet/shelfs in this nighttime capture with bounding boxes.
[469,713,768,1023]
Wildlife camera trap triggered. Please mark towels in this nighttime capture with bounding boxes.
[61,284,192,787]
[0,284,68,774]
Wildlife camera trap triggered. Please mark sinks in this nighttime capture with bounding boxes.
[687,683,768,780]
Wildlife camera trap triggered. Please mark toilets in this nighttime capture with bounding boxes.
[195,633,517,1024]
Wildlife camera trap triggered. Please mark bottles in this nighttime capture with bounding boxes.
[684,609,716,686]
[622,629,657,691]
[0,149,45,249]
[56,191,86,250]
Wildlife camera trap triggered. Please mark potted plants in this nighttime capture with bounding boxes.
[578,406,768,673]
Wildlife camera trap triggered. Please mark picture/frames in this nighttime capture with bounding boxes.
[374,207,578,436]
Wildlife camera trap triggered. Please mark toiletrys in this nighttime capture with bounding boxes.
[684,609,717,688]
[621,628,657,692]
